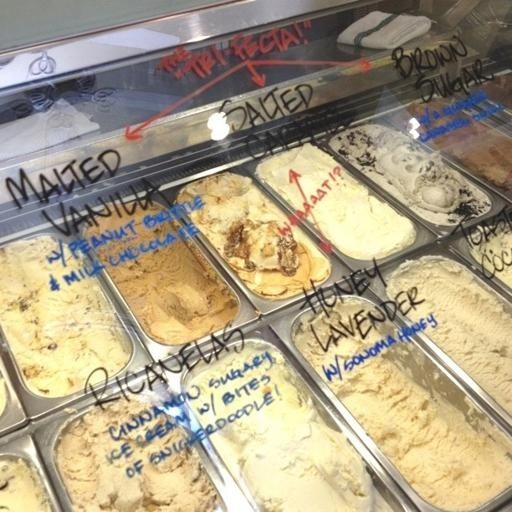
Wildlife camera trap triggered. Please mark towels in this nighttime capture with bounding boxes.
[0,95,105,165]
[334,7,434,54]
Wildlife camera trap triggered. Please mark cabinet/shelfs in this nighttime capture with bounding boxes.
[0,0,511,512]
